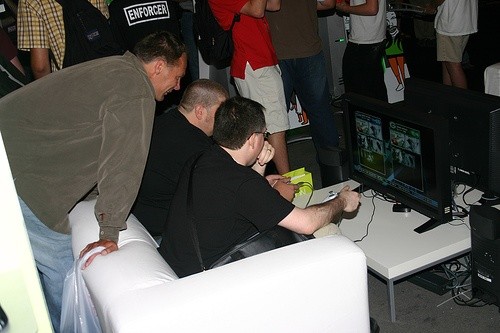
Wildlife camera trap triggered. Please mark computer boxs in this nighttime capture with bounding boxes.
[471,231,500,312]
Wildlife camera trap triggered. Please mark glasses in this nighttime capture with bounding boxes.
[247,128,268,138]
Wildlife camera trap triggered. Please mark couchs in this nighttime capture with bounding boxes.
[68,196,370,333]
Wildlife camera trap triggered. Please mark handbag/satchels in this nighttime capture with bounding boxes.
[60,246,106,333]
[209,225,308,269]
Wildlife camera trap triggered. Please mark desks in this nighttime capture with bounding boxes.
[307,179,500,323]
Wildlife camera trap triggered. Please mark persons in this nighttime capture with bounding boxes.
[132,79,234,238]
[155,95,380,333]
[0,30,188,321]
[0,0,478,174]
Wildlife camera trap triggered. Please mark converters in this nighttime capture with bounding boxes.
[407,271,454,297]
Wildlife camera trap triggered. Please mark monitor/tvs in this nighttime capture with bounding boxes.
[405,77,500,206]
[347,91,454,233]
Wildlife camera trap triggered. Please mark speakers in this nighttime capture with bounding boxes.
[319,146,346,166]
[469,205,500,240]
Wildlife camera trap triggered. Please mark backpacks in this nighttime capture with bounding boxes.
[192,0,241,70]
[55,0,123,69]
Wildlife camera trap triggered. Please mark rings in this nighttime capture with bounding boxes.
[267,148,271,153]
